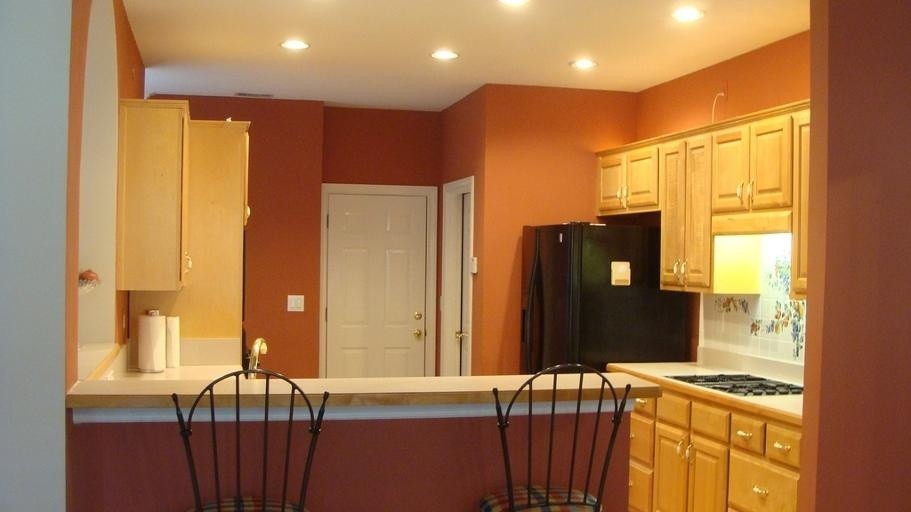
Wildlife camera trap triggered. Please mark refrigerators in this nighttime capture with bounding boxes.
[518,222,694,374]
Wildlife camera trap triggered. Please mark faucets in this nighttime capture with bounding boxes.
[247,337,268,379]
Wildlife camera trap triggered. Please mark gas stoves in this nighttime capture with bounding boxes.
[662,372,805,398]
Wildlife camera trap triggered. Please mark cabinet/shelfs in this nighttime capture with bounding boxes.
[118,99,250,368]
